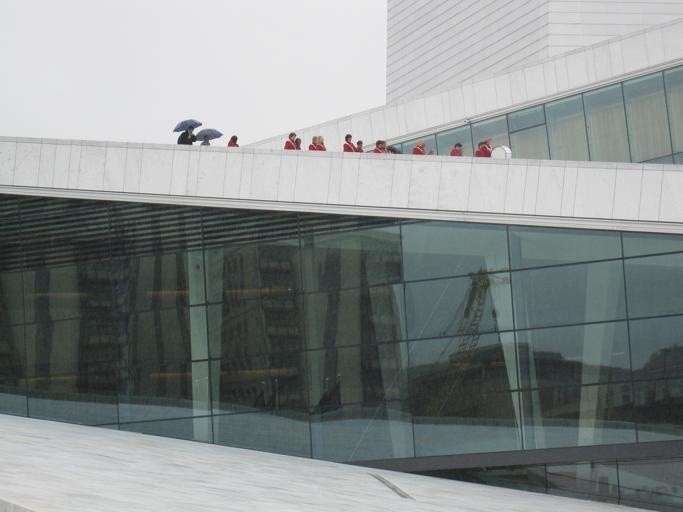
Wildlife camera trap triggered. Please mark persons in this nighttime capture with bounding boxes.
[199,134,210,145]
[226,135,240,146]
[176,125,195,145]
[283,131,494,157]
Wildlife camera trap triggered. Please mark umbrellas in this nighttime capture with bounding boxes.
[172,118,201,137]
[195,128,222,142]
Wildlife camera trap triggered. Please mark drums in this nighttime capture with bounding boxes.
[491,146,512,159]
